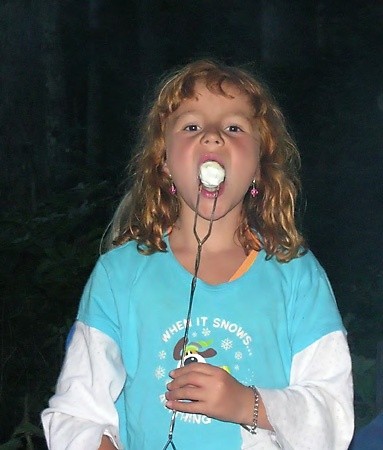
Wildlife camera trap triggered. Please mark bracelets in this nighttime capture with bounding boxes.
[239,386,260,437]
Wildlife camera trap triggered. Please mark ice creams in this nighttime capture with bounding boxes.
[200,162,226,187]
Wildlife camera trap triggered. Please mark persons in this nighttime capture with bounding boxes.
[39,56,356,449]
